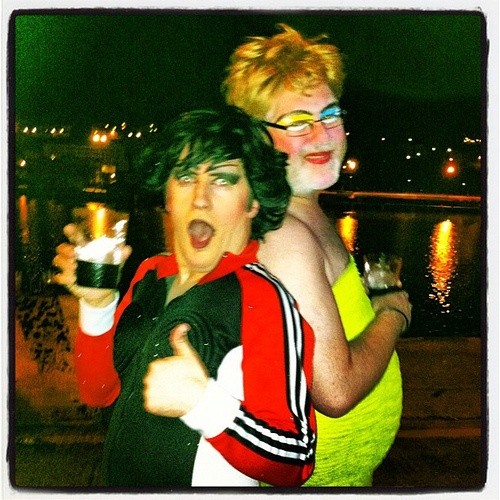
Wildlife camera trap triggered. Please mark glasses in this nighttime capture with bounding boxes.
[260,108,349,137]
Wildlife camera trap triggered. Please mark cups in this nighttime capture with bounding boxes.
[73,207,130,291]
[362,253,402,299]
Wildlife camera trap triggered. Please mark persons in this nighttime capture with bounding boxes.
[222,24,414,487]
[54,103,316,486]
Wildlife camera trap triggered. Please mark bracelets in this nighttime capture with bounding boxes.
[376,307,408,332]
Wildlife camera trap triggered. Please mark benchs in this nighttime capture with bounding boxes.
[14,323,483,447]
